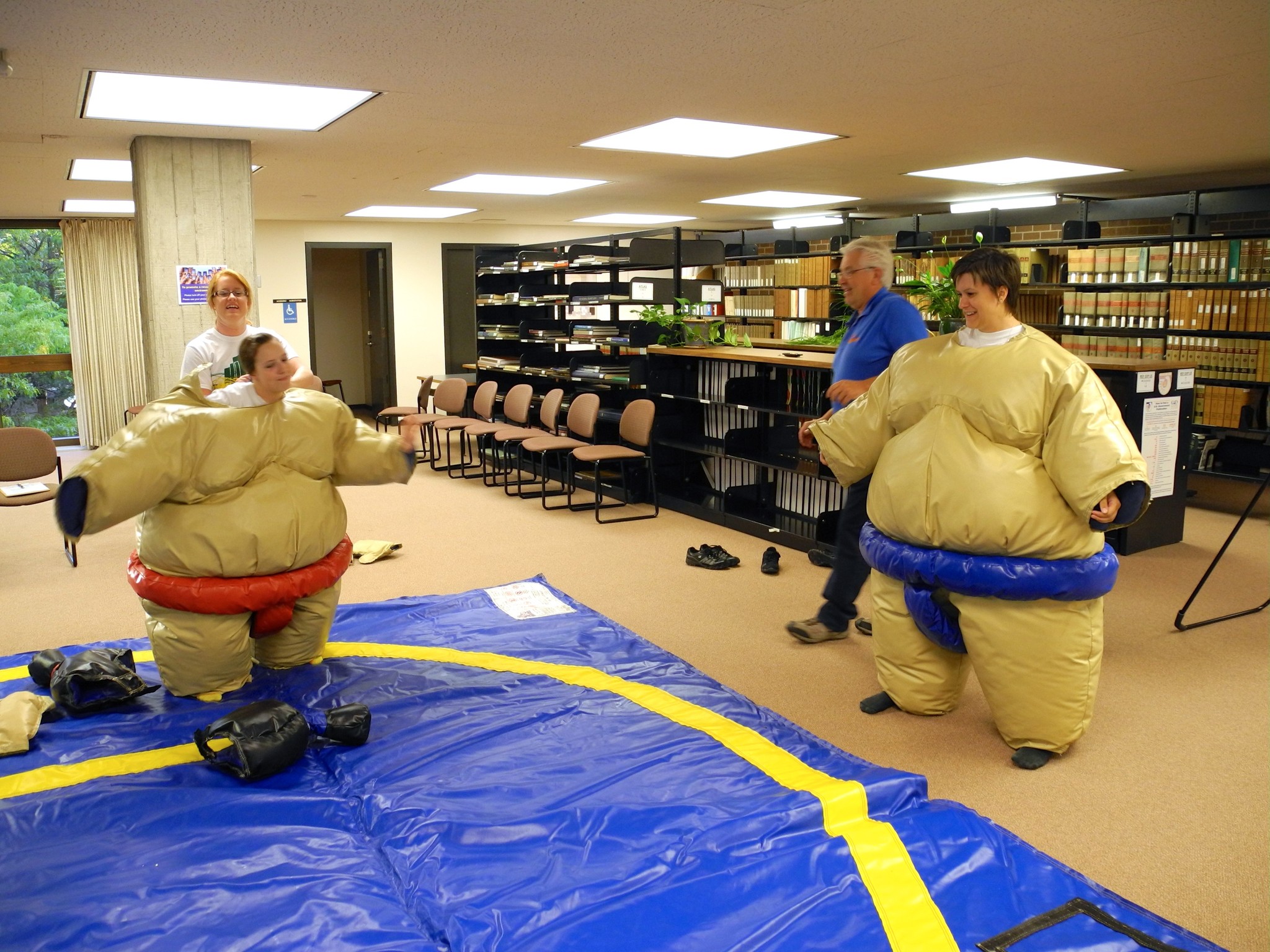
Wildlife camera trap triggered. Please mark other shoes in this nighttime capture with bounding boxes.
[807,548,835,568]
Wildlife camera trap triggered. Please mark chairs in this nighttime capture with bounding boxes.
[375,376,659,524]
[0,427,77,568]
[322,380,346,403]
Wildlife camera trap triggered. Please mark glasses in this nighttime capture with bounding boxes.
[211,290,249,297]
[836,266,876,280]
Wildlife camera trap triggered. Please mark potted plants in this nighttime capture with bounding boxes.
[638,298,753,349]
[892,230,984,335]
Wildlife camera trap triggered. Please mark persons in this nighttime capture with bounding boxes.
[786,238,929,645]
[180,269,300,394]
[808,247,1152,770]
[55,329,417,701]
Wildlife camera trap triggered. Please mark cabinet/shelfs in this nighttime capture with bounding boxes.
[475,193,1270,555]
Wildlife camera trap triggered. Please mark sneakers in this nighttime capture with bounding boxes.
[686,545,728,570]
[703,544,740,567]
[855,617,872,636]
[761,546,781,574]
[785,617,849,643]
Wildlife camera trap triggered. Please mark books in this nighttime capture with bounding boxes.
[475,293,569,303]
[572,294,630,301]
[477,255,631,272]
[692,305,725,315]
[477,325,629,342]
[477,356,630,381]
